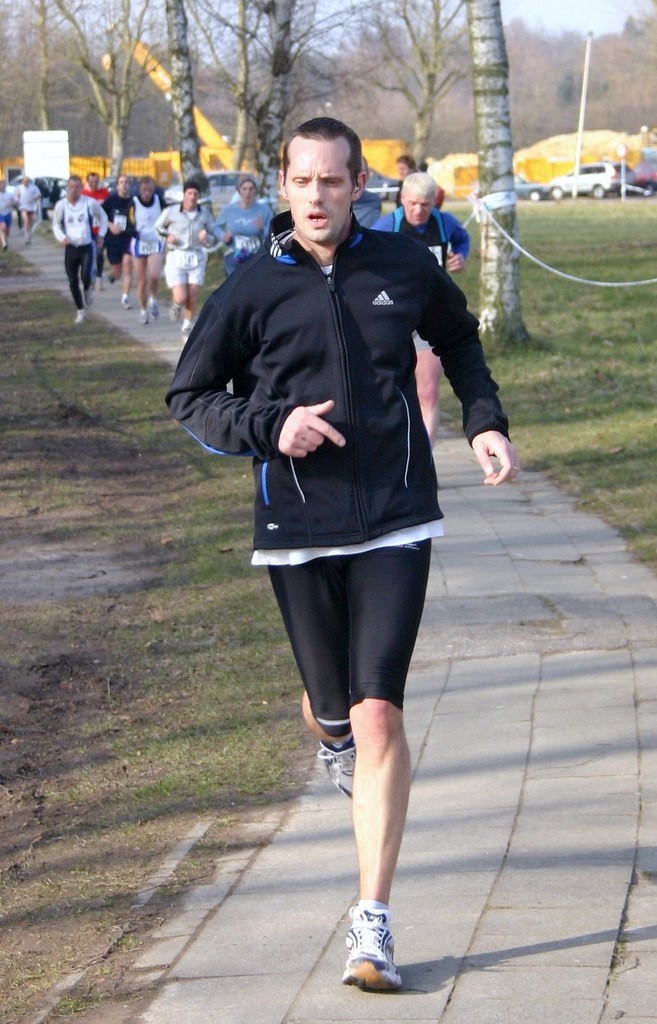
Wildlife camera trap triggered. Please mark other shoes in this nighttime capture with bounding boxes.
[170,306,181,323]
[121,298,132,310]
[150,298,160,320]
[74,306,88,324]
[25,237,31,244]
[83,287,94,305]
[96,276,105,291]
[108,274,114,283]
[140,312,148,325]
[180,318,192,332]
[3,241,8,252]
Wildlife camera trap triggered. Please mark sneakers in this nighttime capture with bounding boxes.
[318,738,357,799]
[343,904,401,990]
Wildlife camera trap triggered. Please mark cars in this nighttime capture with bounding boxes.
[162,168,260,205]
[5,174,68,220]
[543,160,635,200]
[354,166,402,204]
[101,175,166,200]
[628,161,657,194]
[512,172,546,202]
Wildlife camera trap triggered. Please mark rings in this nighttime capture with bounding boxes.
[514,467,519,471]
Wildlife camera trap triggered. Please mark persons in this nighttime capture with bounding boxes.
[351,152,471,450]
[164,120,521,991]
[0,172,274,344]
